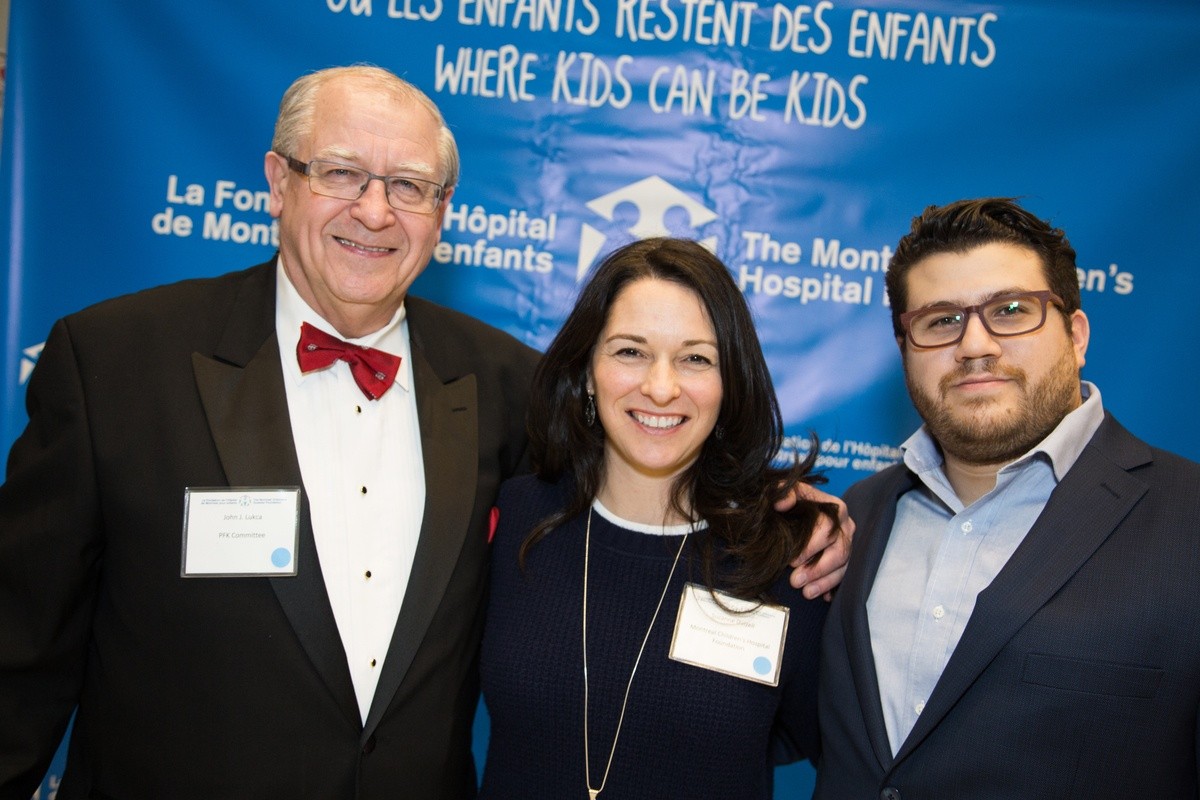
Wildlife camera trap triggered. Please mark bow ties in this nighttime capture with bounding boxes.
[297,323,401,401]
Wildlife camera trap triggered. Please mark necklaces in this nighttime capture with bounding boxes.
[583,501,691,800]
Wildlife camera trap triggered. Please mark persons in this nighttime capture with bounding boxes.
[812,196,1200,800]
[479,238,840,800]
[0,66,855,800]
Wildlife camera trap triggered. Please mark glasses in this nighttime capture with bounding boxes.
[272,152,449,213]
[900,288,1070,349]
